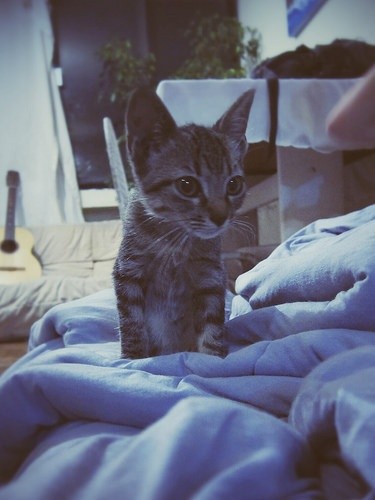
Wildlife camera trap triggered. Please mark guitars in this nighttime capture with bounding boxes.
[0,171,43,285]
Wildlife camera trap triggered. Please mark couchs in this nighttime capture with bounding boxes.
[0,220,128,341]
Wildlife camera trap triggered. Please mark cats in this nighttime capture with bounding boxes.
[111,83,262,361]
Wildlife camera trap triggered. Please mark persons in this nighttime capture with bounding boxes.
[325,64,375,147]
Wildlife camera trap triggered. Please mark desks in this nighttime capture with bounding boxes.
[155,79,375,243]
